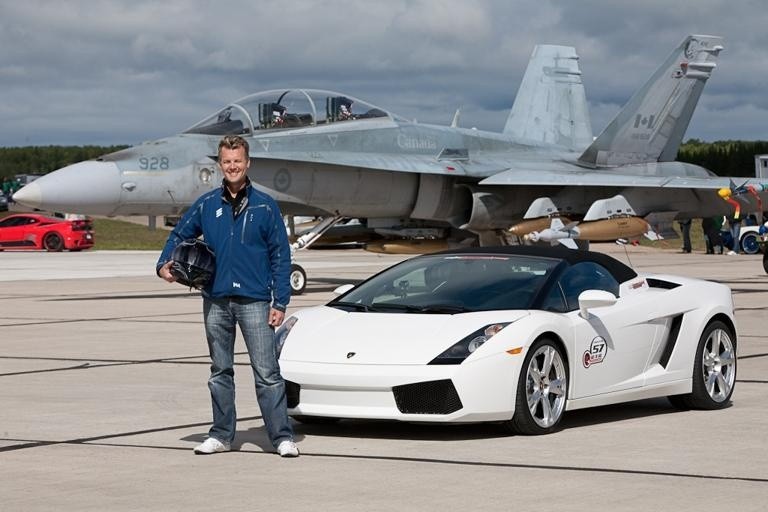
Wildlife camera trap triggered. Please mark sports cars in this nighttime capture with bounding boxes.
[277,247,739,435]
[1,213,93,251]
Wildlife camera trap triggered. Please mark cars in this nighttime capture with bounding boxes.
[737,222,768,255]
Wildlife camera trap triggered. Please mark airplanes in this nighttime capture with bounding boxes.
[11,34,768,273]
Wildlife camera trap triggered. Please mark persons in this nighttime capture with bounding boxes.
[155,134,301,459]
[678,211,768,256]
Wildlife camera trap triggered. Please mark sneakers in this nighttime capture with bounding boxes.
[193,435,231,454]
[275,440,298,457]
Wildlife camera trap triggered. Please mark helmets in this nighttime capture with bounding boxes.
[168,237,217,298]
